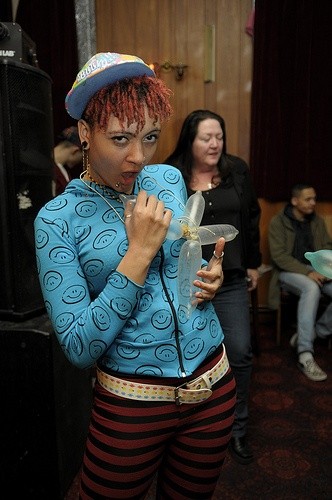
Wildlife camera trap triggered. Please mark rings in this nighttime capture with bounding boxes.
[125,215,131,218]
[213,251,224,259]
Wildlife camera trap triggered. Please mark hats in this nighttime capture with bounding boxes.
[62,126,81,148]
[64,52,155,120]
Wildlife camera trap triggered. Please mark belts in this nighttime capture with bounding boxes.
[94,340,231,407]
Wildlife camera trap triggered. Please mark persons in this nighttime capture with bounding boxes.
[53,127,83,198]
[269,186,332,380]
[34,52,235,500]
[164,109,263,463]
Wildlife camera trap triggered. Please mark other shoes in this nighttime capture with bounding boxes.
[298,359,327,382]
[291,326,317,350]
[228,437,253,463]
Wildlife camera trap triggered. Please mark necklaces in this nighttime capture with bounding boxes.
[88,170,141,204]
